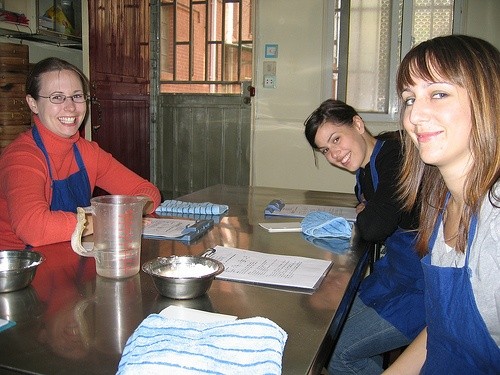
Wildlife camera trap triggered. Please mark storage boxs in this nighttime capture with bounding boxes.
[0,43,29,63]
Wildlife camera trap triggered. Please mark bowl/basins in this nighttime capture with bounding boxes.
[142,256,225,299]
[0,286,46,322]
[150,293,215,316]
[0,249,44,293]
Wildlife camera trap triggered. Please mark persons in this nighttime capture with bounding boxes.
[376,34,500,375]
[305,99,427,375]
[0,57,161,253]
[29,241,96,367]
[301,251,362,330]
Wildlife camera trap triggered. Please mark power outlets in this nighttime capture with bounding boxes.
[263,62,276,89]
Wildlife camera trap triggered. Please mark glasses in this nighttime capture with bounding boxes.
[32,94,88,104]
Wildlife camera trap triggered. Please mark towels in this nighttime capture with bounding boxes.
[301,233,351,256]
[114,312,289,374]
[299,211,355,239]
[155,199,229,215]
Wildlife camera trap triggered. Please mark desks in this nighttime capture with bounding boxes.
[0,183,379,375]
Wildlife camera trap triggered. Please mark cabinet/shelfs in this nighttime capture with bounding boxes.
[0,64,34,155]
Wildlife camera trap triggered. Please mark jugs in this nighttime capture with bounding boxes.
[70,194,149,280]
[74,274,144,355]
